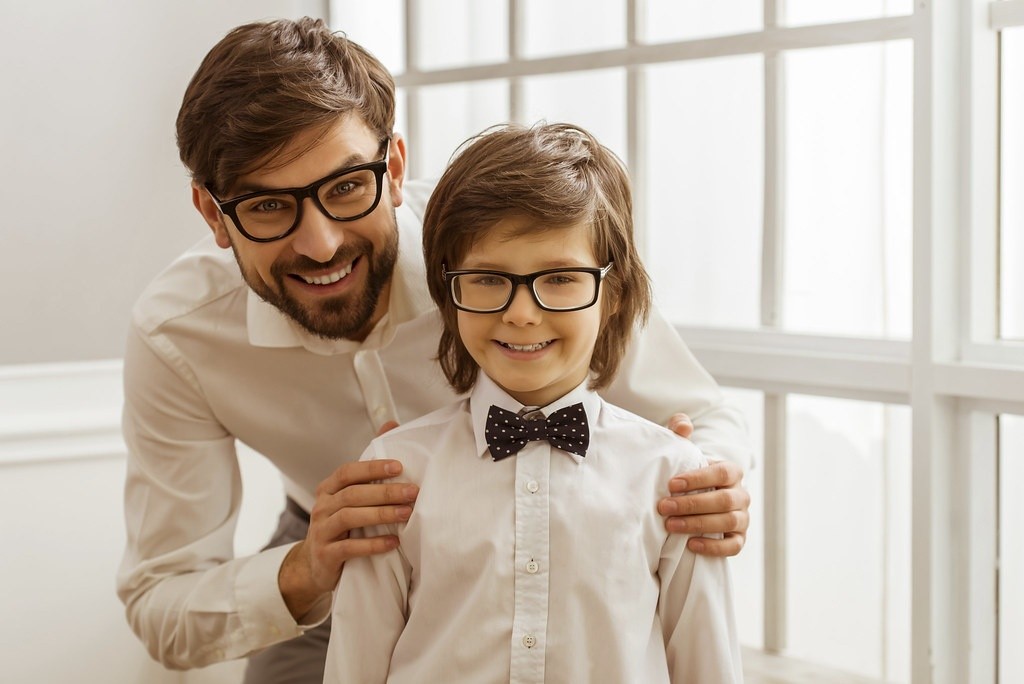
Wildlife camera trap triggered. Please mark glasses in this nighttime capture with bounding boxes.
[442,261,612,313]
[204,139,391,242]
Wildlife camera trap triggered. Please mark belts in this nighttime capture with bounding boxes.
[287,496,312,524]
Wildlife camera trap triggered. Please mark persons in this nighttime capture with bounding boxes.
[320,120,747,684]
[116,17,751,684]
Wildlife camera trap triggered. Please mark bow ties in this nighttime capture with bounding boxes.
[486,407,591,462]
